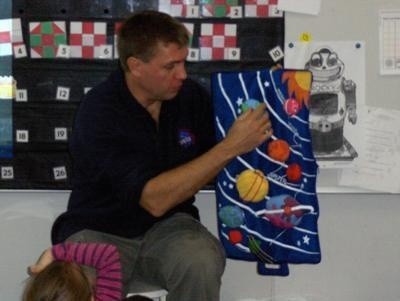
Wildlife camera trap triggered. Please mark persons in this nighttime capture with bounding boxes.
[20,242,123,300]
[52,9,273,301]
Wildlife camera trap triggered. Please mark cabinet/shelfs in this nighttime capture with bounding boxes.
[0,0,286,192]
